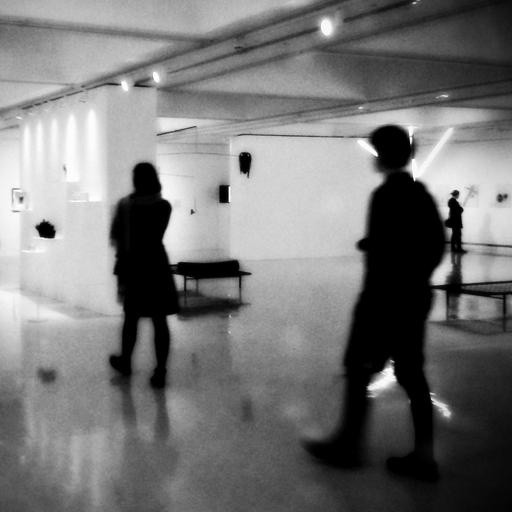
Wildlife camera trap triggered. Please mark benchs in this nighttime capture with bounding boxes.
[173,261,252,293]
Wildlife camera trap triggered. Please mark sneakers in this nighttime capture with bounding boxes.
[304,437,362,465]
[387,450,438,482]
[110,355,131,375]
[151,369,165,389]
[451,246,465,253]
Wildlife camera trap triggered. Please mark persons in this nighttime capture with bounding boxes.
[448,190,468,253]
[303,125,445,481]
[109,163,181,388]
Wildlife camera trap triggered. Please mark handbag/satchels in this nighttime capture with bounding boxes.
[445,218,452,228]
